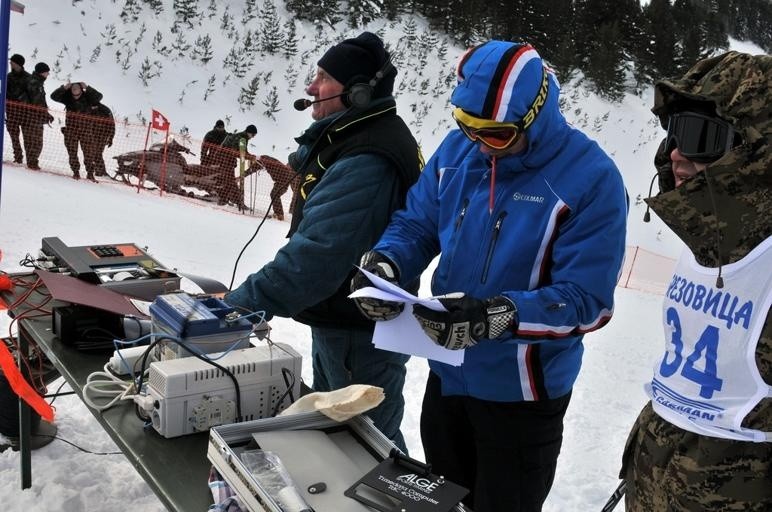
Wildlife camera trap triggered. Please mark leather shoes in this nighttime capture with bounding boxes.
[73,170,80,178]
[87,172,95,182]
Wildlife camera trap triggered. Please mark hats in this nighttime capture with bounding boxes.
[11,54,25,67]
[247,125,258,134]
[35,62,50,74]
[317,31,397,103]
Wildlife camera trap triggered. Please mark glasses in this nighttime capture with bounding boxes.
[451,108,522,153]
[663,111,736,162]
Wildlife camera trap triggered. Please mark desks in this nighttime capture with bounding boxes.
[0,272,472,512]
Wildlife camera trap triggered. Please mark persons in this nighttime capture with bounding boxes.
[51,81,102,183]
[28,63,54,169]
[8,54,30,164]
[214,33,422,461]
[215,124,258,210]
[620,50,771,512]
[237,155,298,221]
[84,103,115,176]
[351,39,629,512]
[200,120,227,164]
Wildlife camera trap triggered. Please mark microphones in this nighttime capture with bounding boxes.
[293,90,346,112]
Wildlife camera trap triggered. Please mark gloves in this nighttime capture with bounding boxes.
[412,291,518,351]
[350,250,402,321]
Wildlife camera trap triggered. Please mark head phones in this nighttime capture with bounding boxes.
[341,53,397,108]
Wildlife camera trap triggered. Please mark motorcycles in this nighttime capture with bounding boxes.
[112,134,221,202]
